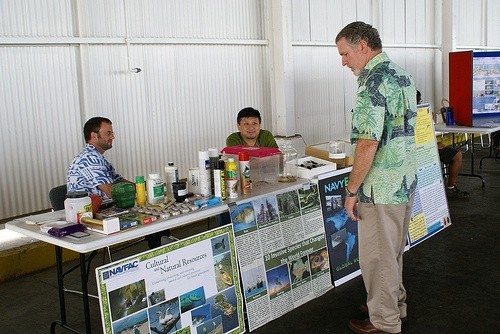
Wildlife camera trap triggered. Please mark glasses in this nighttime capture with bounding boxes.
[105,132,115,136]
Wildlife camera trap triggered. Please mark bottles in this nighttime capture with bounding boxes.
[198,148,251,202]
[64,191,93,223]
[148,175,167,204]
[172,181,186,204]
[279,143,299,182]
[180,178,191,194]
[135,174,147,208]
[164,162,180,194]
[446,107,453,125]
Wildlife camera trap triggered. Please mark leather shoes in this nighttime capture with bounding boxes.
[359,302,408,326]
[347,318,405,334]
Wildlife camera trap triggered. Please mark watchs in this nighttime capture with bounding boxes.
[345,187,359,198]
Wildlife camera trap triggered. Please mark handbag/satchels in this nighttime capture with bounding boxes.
[440,98,454,126]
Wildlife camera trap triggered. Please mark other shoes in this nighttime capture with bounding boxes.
[446,185,469,199]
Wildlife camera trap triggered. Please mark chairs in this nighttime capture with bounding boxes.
[48,184,114,300]
[272,133,309,155]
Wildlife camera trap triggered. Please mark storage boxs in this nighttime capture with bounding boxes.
[304,139,354,167]
[221,145,282,184]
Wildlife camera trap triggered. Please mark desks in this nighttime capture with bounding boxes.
[5,118,500,334]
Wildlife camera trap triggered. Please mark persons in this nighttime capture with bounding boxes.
[67,117,171,250]
[416,89,469,200]
[226,107,280,148]
[335,21,418,334]
